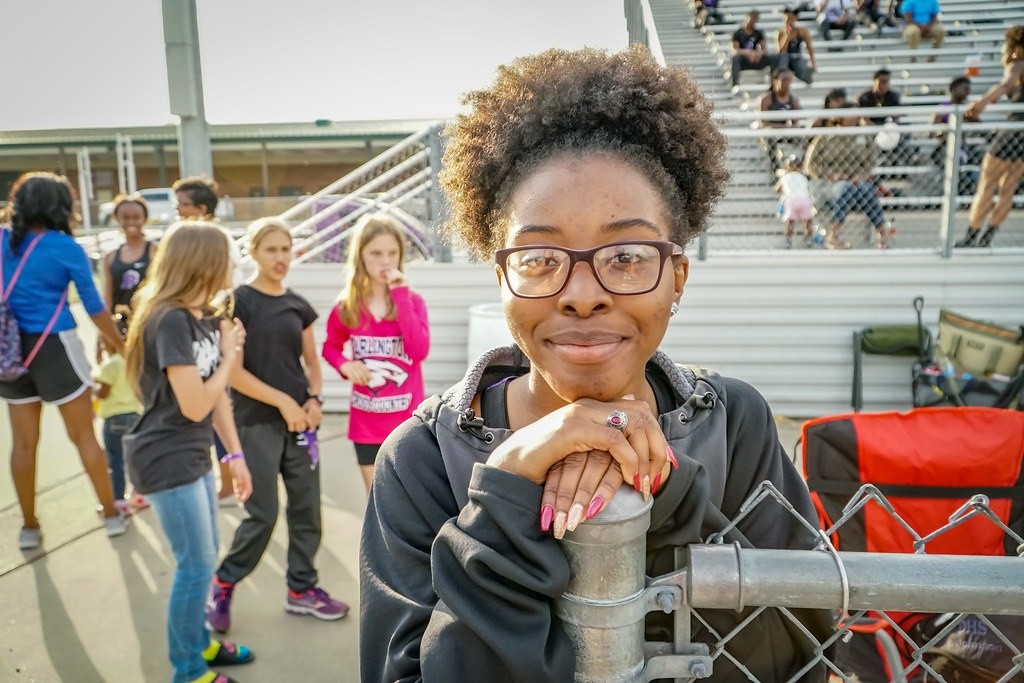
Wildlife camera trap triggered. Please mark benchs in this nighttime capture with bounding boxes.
[691,0,1024,208]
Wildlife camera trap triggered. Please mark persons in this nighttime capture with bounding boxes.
[815,0,904,52]
[165,178,247,508]
[770,7,817,85]
[899,0,943,64]
[772,155,818,249]
[804,99,894,249]
[359,47,836,683]
[953,24,1024,248]
[757,67,1018,207]
[203,218,350,634]
[91,304,151,519]
[322,214,430,497]
[102,193,159,319]
[731,9,785,90]
[695,0,732,35]
[124,221,256,683]
[0,171,131,549]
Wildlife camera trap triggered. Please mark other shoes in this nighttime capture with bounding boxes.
[184,669,240,683]
[824,239,850,249]
[96,500,131,515]
[129,489,149,507]
[203,640,254,666]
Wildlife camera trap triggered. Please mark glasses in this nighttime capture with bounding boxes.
[494,241,681,300]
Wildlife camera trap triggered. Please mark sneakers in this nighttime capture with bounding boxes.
[19,527,39,549]
[104,510,127,537]
[206,574,234,632]
[286,587,351,621]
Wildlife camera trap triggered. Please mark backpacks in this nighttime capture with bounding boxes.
[0,229,70,382]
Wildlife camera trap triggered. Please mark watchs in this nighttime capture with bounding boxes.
[309,394,323,406]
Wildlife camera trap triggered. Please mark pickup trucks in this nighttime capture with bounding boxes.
[98,188,236,228]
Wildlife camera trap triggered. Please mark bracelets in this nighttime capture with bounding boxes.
[220,453,245,464]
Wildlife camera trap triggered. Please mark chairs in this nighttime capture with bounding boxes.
[911,308,1023,410]
[798,406,1024,683]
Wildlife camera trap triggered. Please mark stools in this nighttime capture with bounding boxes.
[852,321,934,410]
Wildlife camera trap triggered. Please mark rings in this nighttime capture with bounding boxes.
[608,409,628,430]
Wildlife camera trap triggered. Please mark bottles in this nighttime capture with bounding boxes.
[776,200,787,222]
[813,220,825,248]
[887,218,897,246]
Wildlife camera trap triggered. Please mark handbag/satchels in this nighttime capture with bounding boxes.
[938,311,1024,378]
